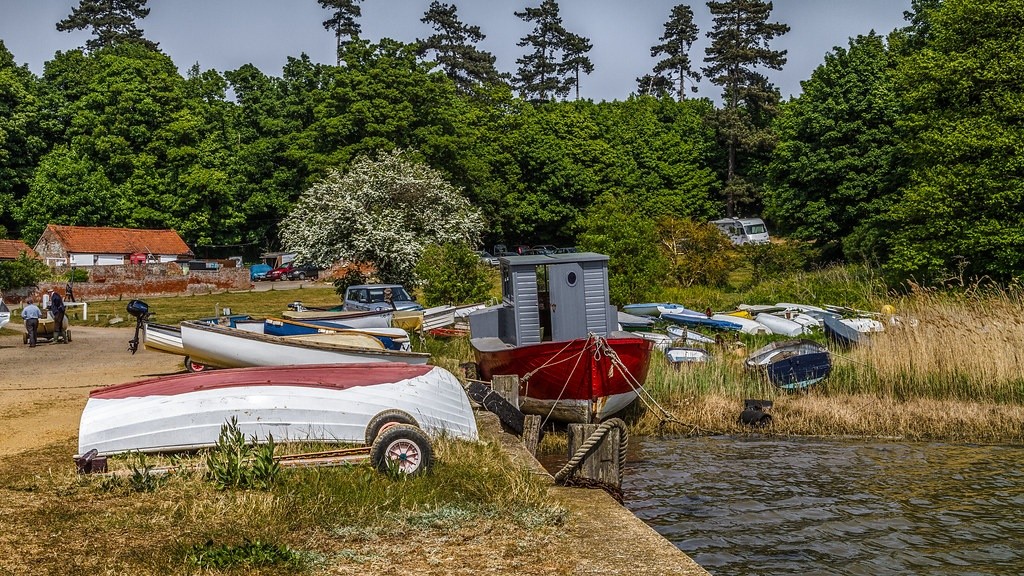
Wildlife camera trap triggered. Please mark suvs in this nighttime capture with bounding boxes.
[343,284,424,314]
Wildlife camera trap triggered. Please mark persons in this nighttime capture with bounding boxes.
[45,286,69,345]
[21,297,43,347]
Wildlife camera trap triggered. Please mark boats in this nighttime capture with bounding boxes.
[417,304,457,331]
[74,362,479,484]
[452,303,485,320]
[744,339,833,391]
[667,323,716,351]
[468,251,656,423]
[624,301,684,320]
[181,307,431,373]
[631,330,671,355]
[234,306,394,335]
[429,320,471,341]
[711,302,920,350]
[664,347,716,373]
[262,315,410,352]
[287,300,424,333]
[660,308,773,336]
[26,310,68,334]
[126,299,188,357]
[617,310,656,335]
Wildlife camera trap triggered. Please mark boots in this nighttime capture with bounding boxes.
[59,332,68,344]
[49,332,59,345]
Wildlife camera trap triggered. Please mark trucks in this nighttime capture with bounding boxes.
[709,217,769,245]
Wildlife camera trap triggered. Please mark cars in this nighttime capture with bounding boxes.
[249,264,275,282]
[265,261,301,281]
[287,261,331,281]
